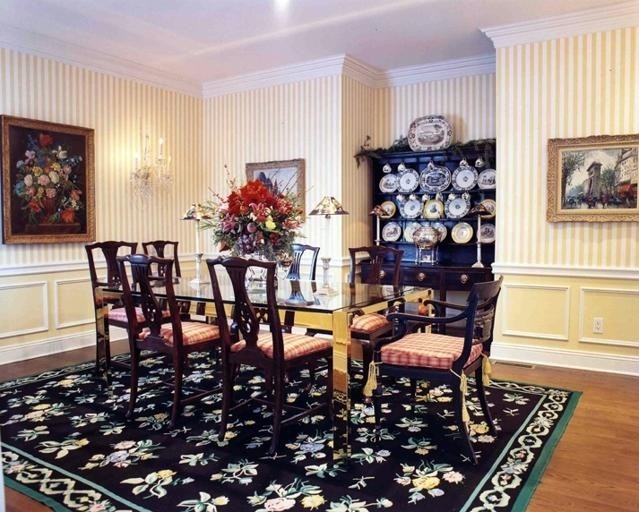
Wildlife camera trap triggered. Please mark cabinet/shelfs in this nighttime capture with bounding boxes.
[367,144,497,333]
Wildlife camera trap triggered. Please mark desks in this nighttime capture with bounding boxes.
[94,279,436,456]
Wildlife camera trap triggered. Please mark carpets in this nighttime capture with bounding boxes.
[0,349,584,512]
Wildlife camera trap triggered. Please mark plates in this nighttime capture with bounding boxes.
[378,115,496,244]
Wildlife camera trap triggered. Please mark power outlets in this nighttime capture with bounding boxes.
[593,317,605,333]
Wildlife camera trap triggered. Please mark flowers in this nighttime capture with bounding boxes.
[180,163,305,262]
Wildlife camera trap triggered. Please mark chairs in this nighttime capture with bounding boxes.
[84,240,168,389]
[115,253,231,431]
[141,240,194,326]
[280,243,320,281]
[372,275,504,465]
[204,256,333,457]
[344,246,404,386]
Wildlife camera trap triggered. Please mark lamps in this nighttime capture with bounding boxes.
[180,203,215,287]
[129,132,172,201]
[306,195,350,297]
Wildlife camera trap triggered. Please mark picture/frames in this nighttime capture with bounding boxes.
[546,134,639,223]
[0,114,97,244]
[245,158,306,223]
